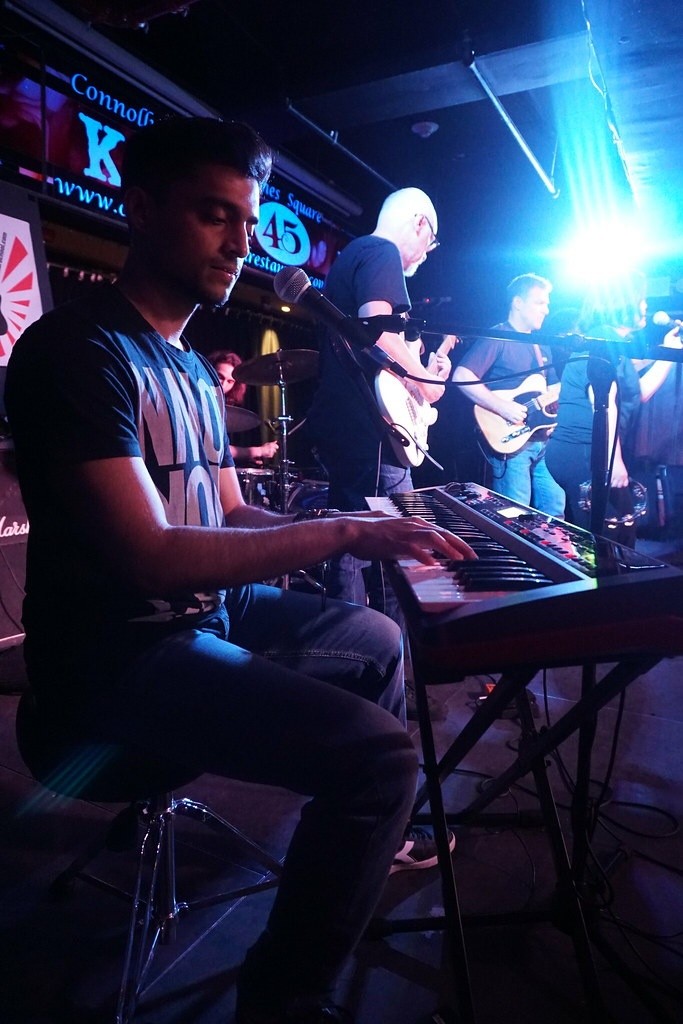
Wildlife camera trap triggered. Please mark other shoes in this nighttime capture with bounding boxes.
[406,678,448,720]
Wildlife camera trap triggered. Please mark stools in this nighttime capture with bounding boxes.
[16,692,284,1024]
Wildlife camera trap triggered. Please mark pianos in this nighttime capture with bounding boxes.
[363,481,681,690]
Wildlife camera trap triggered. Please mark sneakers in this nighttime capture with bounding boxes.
[236,961,354,1024]
[388,824,455,876]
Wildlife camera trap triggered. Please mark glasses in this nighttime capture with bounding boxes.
[414,213,440,253]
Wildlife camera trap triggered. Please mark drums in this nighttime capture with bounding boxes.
[235,467,275,509]
[287,478,330,513]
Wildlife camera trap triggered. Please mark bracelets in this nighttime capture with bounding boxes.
[293,509,345,562]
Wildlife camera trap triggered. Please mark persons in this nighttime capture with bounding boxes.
[206,350,280,465]
[3,117,479,1024]
[307,188,453,722]
[451,270,683,553]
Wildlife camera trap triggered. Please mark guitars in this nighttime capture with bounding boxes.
[372,334,460,469]
[470,372,562,463]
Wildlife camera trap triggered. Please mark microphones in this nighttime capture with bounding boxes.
[653,311,683,336]
[273,267,407,380]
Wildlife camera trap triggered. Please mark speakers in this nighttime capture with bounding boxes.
[263,418,275,436]
[0,437,36,651]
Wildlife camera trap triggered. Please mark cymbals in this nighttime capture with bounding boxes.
[224,403,262,434]
[230,347,321,387]
[578,476,649,531]
